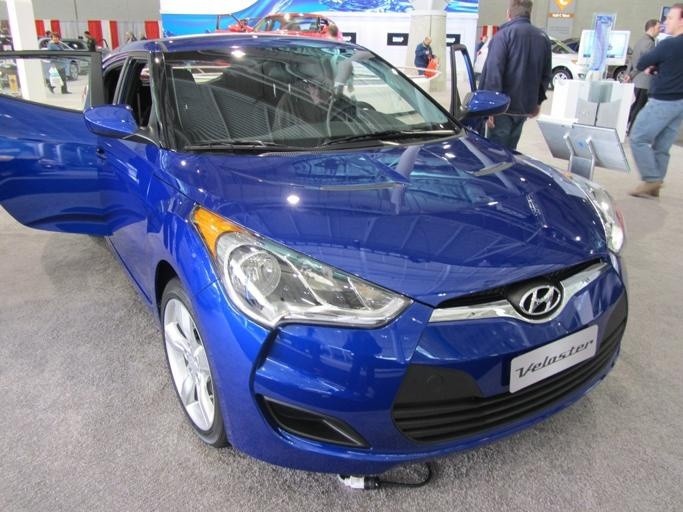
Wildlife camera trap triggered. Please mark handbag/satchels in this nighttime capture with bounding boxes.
[424,57,438,77]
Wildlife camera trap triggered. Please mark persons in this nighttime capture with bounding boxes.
[414,37,436,75]
[44,31,139,94]
[623,20,661,135]
[629,4,683,197]
[478,0,552,152]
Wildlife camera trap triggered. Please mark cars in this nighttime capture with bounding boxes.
[0,32,83,84]
[556,35,635,80]
[0,37,626,478]
[473,34,592,85]
[212,11,343,64]
[57,37,111,74]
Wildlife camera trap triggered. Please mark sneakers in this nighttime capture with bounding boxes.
[630,180,662,197]
